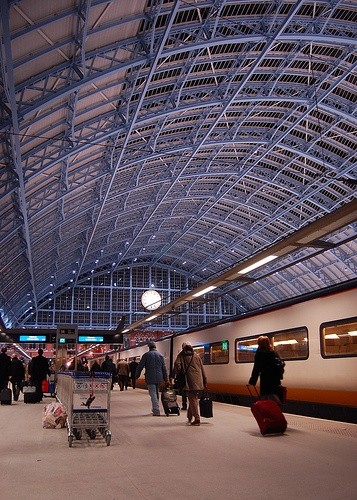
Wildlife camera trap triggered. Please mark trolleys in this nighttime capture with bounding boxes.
[52,370,113,448]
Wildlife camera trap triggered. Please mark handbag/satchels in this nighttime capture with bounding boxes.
[266,351,286,373]
[278,385,287,403]
[174,374,186,387]
[43,401,67,429]
[199,386,213,418]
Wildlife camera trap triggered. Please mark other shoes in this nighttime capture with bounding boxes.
[181,407,187,410]
[151,409,160,416]
[183,417,200,426]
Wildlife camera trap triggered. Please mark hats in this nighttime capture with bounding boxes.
[38,348,43,354]
[182,343,188,349]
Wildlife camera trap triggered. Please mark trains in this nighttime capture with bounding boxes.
[80,276,357,423]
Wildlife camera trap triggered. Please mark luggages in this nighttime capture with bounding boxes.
[1,379,12,405]
[246,383,287,436]
[161,384,180,416]
[24,379,36,404]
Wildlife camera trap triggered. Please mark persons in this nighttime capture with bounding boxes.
[174,345,207,427]
[68,353,139,391]
[0,346,13,395]
[9,354,25,402]
[170,342,196,410]
[135,340,168,417]
[13,349,50,401]
[248,335,286,417]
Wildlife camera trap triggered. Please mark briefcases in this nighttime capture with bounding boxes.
[41,378,55,393]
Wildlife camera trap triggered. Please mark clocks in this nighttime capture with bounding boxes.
[142,291,161,310]
